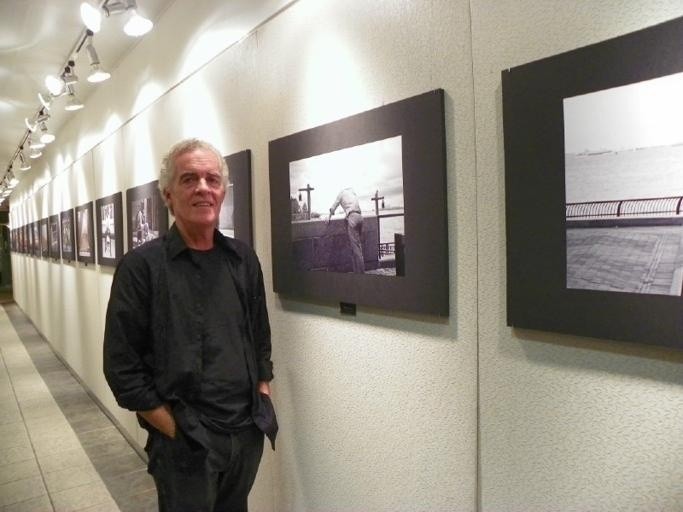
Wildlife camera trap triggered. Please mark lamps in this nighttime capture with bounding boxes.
[0,1,154,211]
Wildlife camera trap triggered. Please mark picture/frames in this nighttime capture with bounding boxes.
[266,87,451,317]
[500,16,683,351]
[8,149,255,271]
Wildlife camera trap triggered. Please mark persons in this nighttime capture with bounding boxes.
[137,211,143,243]
[142,216,150,242]
[105,209,111,256]
[102,135,278,510]
[325,183,367,273]
